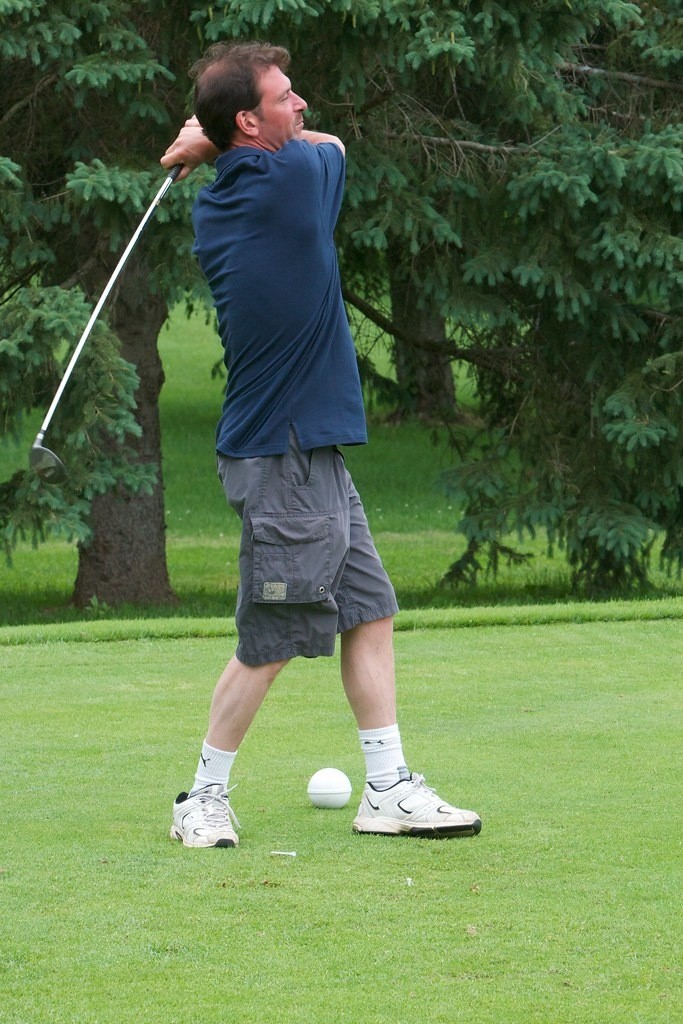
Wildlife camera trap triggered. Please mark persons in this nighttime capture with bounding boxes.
[160,43,482,849]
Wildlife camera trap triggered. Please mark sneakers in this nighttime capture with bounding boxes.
[170,783,242,848]
[352,771,482,838]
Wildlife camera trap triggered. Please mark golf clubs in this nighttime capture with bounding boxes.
[28,162,186,484]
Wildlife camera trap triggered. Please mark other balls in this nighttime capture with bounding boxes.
[306,767,354,810]
